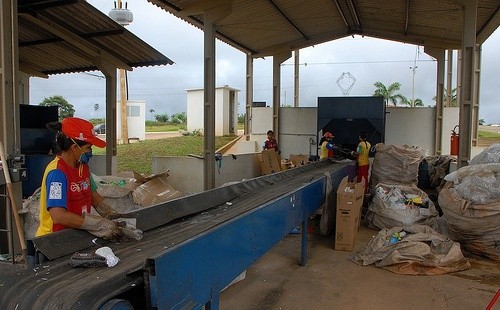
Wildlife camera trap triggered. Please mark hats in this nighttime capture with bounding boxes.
[324,132,334,138]
[62,117,108,147]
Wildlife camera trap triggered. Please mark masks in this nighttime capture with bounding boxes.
[329,139,332,143]
[73,147,93,164]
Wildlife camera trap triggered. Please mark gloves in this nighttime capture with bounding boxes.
[351,151,355,156]
[80,213,130,243]
[96,200,120,221]
[277,150,281,155]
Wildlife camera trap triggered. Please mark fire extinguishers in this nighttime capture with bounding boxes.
[448,125,459,155]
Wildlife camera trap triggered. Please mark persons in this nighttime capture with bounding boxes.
[260,130,281,161]
[350,135,371,194]
[319,132,342,162]
[39,122,62,156]
[34,117,127,249]
